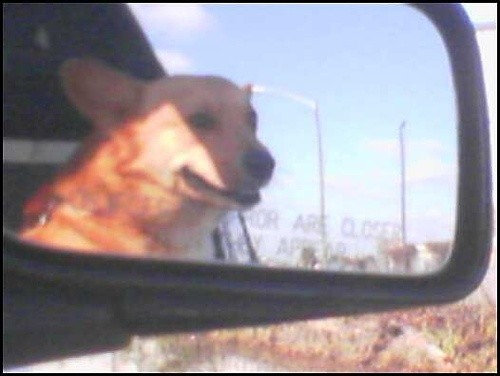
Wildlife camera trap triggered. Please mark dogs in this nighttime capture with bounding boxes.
[17,53,276,259]
